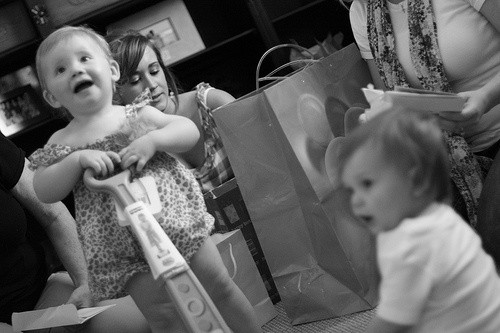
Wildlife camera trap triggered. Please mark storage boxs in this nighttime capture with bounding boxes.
[204,175,281,305]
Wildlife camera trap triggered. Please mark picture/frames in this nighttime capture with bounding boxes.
[0,83,52,138]
[106,0,205,68]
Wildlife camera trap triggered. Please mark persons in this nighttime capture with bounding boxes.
[27,26,266,333]
[339,105,500,333]
[348,0,500,238]
[106,33,238,141]
[0,127,149,333]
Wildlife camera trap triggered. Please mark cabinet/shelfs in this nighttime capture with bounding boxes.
[0,0,355,145]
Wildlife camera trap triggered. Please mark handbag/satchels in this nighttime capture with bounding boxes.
[212,230,278,333]
[212,41,378,327]
